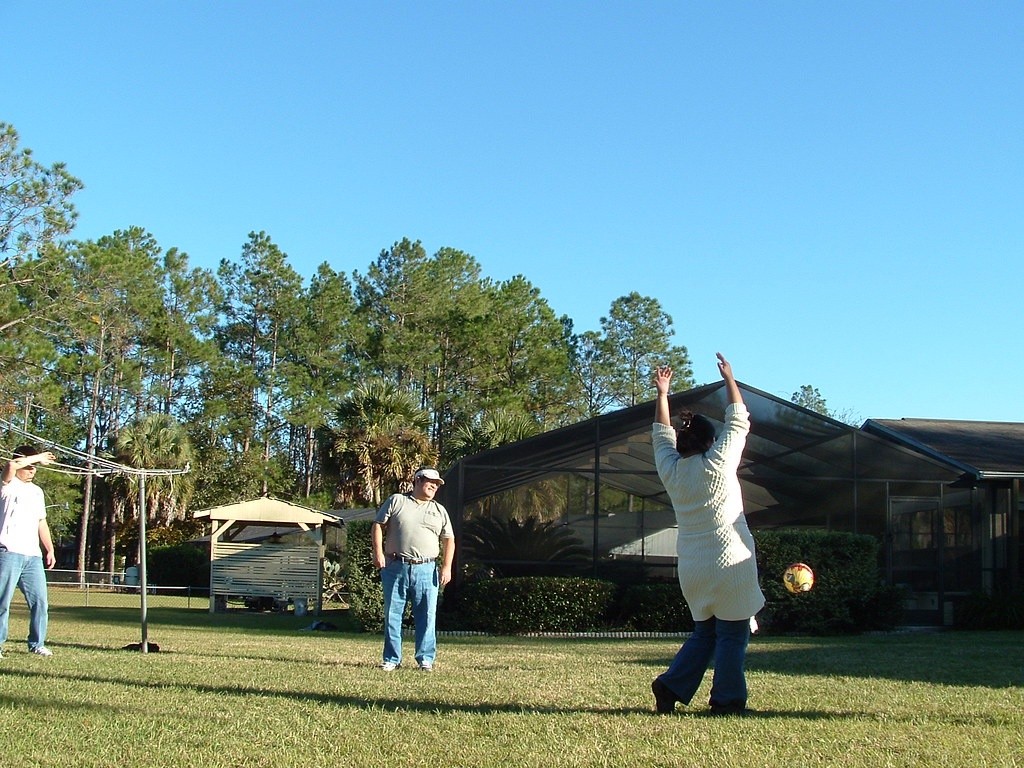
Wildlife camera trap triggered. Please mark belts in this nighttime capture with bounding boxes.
[385,552,436,564]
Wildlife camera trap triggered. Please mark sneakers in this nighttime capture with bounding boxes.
[30,646,53,656]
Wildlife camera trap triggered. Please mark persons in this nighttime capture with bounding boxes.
[371,468,456,673]
[650,351,767,714]
[0,445,57,659]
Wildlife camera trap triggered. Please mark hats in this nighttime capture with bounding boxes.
[415,470,444,485]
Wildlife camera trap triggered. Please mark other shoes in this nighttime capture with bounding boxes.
[417,661,432,672]
[652,679,677,713]
[381,662,400,671]
[710,705,753,715]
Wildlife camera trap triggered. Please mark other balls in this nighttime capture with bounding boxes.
[783,563,814,593]
